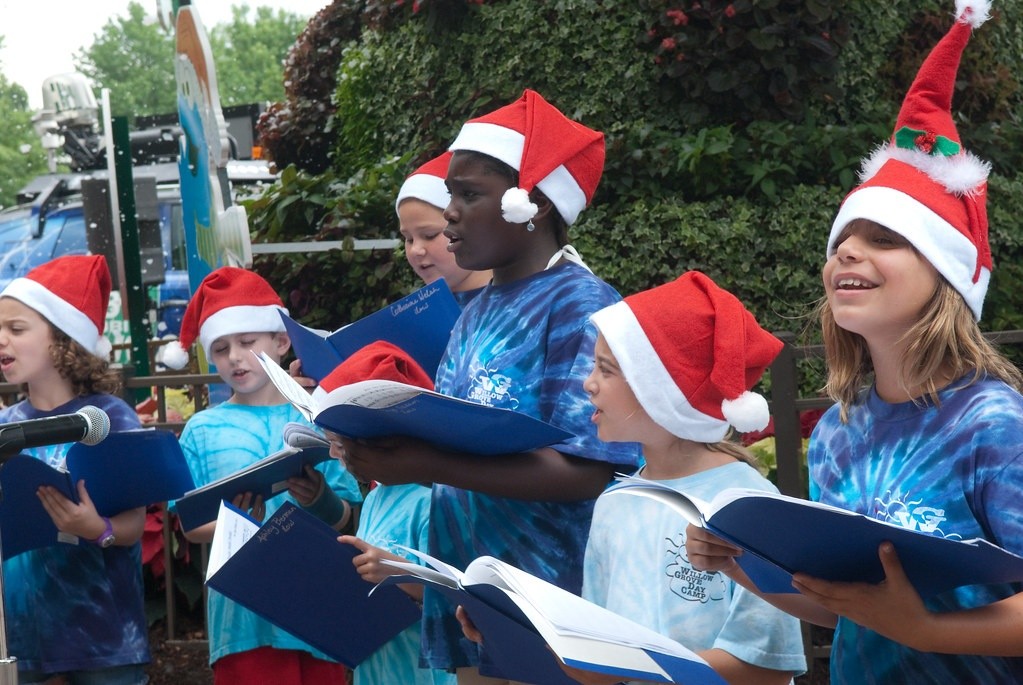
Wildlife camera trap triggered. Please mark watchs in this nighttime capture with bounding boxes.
[88,517,115,548]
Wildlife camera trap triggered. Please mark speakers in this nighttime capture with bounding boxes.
[81,176,165,291]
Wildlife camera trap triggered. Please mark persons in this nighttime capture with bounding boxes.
[0,254,150,685]
[686,151,1022,684]
[396,152,491,293]
[329,88,643,672]
[166,266,365,685]
[455,271,808,685]
[313,341,456,685]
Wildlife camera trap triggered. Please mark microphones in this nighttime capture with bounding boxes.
[0,404,110,454]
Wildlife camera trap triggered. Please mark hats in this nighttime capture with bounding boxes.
[447,89,606,227]
[589,268,784,444]
[826,0,993,322]
[312,340,434,407]
[0,255,113,363]
[395,151,453,218]
[161,266,289,371]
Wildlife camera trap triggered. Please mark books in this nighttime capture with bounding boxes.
[0,429,195,562]
[204,500,422,669]
[176,422,337,534]
[606,471,1023,600]
[279,280,462,384]
[369,544,724,685]
